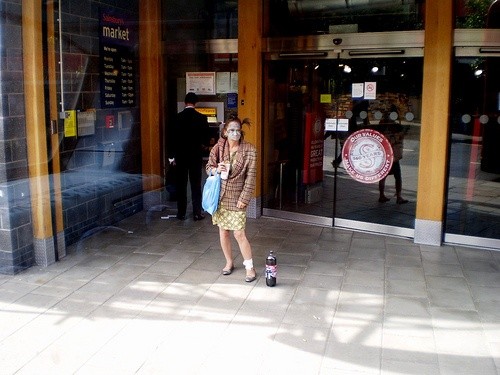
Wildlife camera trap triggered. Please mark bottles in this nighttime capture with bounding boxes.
[266,250,276,287]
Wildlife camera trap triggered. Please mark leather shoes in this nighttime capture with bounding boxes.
[246,269,256,282]
[193,214,205,221]
[223,262,234,274]
[177,213,185,219]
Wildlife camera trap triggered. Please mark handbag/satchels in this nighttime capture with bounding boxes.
[202,168,221,215]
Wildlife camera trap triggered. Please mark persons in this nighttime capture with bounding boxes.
[377,104,408,204]
[201,118,258,282]
[166,92,210,221]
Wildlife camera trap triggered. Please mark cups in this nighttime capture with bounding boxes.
[221,164,230,179]
